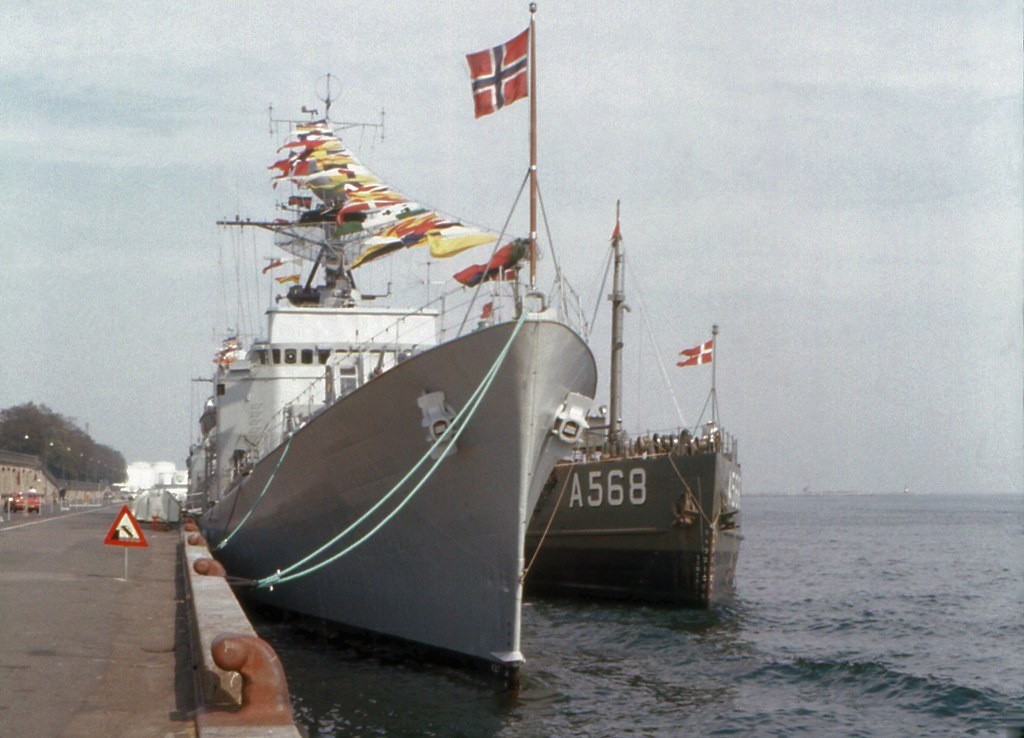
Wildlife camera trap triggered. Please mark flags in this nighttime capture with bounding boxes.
[426,231,499,258]
[676,341,712,366]
[271,219,289,226]
[452,238,524,288]
[262,256,303,272]
[287,194,312,210]
[464,29,529,119]
[269,119,480,272]
[213,336,239,366]
[277,275,300,285]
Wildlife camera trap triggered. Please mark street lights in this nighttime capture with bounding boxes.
[16,428,29,494]
[45,436,123,510]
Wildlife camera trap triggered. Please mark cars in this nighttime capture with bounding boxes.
[5,492,41,514]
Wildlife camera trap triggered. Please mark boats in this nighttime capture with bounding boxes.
[200,67,599,696]
[522,200,744,616]
